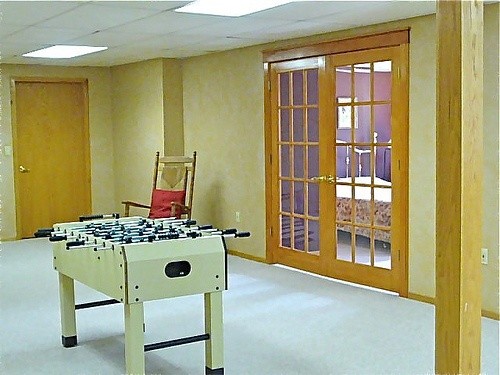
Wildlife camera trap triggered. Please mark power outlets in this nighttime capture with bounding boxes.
[235,211,240,223]
[481,248,488,264]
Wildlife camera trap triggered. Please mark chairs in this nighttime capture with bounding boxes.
[121,150,196,221]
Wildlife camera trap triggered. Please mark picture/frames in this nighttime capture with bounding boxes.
[337,95,359,130]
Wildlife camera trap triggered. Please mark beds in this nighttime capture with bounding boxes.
[336,175,391,246]
